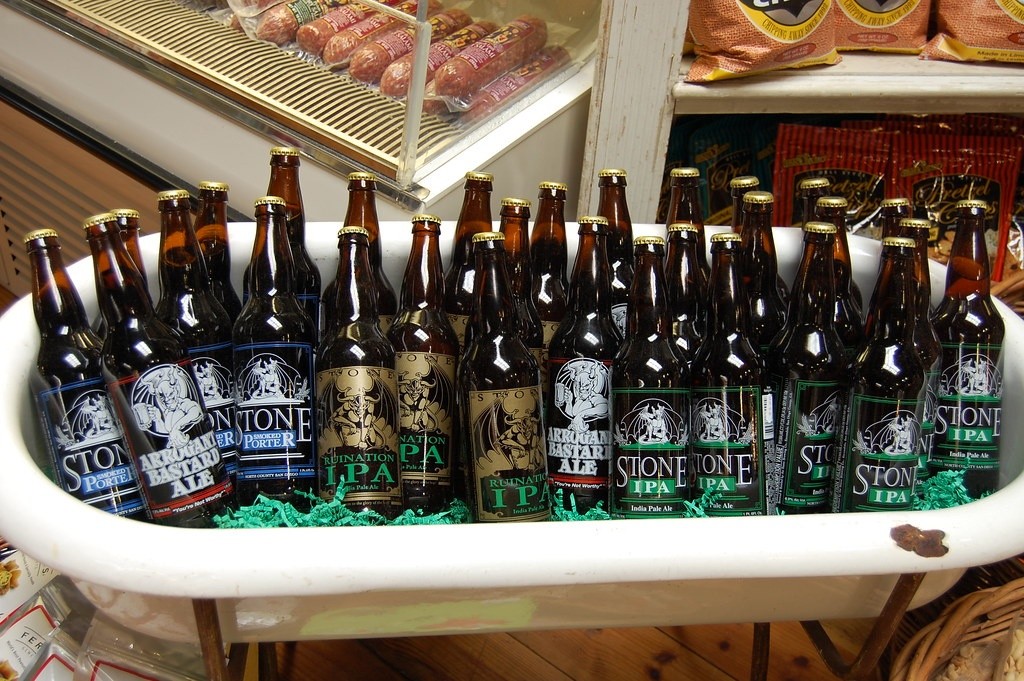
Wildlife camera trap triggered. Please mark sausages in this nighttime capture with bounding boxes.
[228,0,572,123]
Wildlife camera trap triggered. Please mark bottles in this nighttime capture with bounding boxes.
[82,167,941,524]
[23,227,156,525]
[931,199,1005,498]
